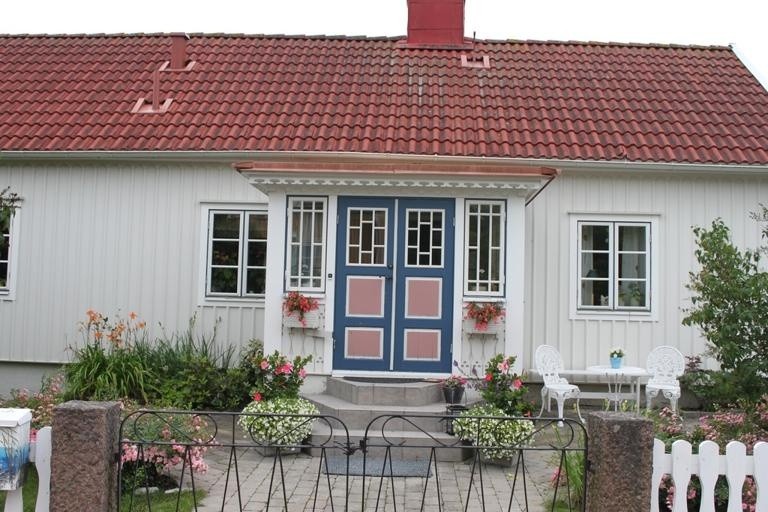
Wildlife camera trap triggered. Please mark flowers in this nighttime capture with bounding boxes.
[606,346,626,357]
[460,299,507,330]
[116,395,223,474]
[236,353,319,443]
[280,288,321,324]
[441,376,466,388]
[441,352,542,457]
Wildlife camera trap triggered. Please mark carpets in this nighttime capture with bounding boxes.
[320,454,431,478]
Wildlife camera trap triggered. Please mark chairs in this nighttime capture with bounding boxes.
[643,346,686,423]
[535,345,586,426]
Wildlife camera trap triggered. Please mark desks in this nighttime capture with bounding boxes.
[590,363,652,413]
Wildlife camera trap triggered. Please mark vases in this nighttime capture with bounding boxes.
[463,320,501,335]
[253,434,304,457]
[119,457,181,495]
[286,311,320,330]
[478,438,517,468]
[612,357,622,368]
[444,388,463,404]
